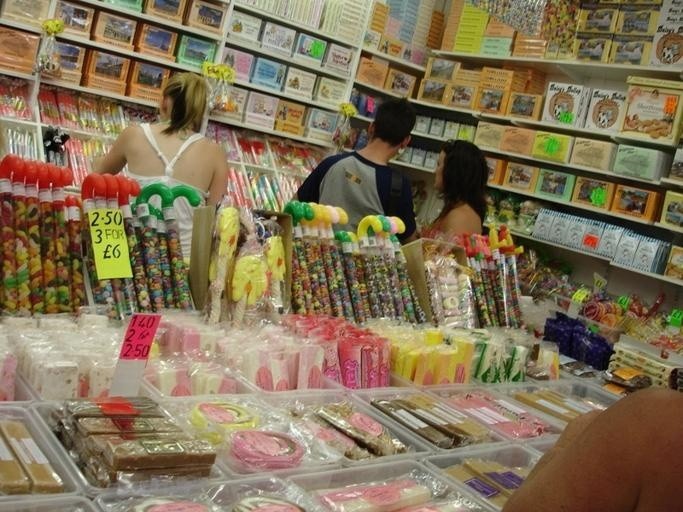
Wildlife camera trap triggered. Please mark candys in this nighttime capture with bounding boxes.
[462,223,521,328]
[284,201,426,325]
[0,155,201,321]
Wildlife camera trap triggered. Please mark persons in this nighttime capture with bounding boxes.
[419,141,488,242]
[289,97,417,245]
[501,389,681,512]
[100,73,228,275]
